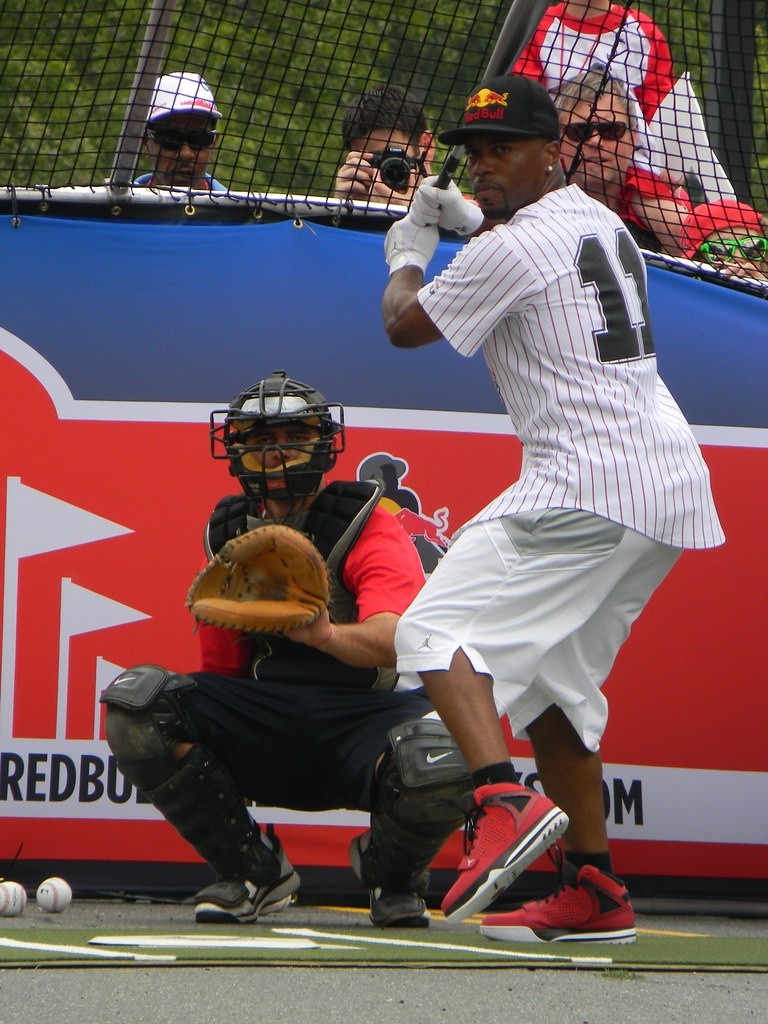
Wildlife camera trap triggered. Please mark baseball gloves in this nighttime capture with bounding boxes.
[187,524,331,631]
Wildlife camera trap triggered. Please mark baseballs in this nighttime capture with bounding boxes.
[37,877,72,913]
[0,881,27,916]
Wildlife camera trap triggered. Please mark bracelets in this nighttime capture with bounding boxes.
[311,624,333,648]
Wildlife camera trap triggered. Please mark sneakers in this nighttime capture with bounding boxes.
[194,823,301,924]
[349,827,432,927]
[440,782,569,926]
[480,844,636,944]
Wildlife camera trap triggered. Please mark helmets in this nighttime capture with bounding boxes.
[210,369,345,526]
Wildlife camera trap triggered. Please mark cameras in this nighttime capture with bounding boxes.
[365,146,418,190]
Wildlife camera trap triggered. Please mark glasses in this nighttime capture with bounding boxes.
[145,125,217,152]
[699,237,767,263]
[560,121,633,143]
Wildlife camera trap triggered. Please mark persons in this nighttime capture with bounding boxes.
[510,0,690,258]
[329,83,438,211]
[130,70,230,191]
[383,72,725,945]
[676,197,767,285]
[101,373,478,930]
[546,63,688,256]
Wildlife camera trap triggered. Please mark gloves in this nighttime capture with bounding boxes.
[409,175,485,237]
[384,213,439,278]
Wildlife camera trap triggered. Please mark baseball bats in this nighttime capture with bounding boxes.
[431,0,547,189]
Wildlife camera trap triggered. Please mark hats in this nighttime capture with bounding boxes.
[147,71,222,121]
[437,73,561,147]
[679,199,764,260]
